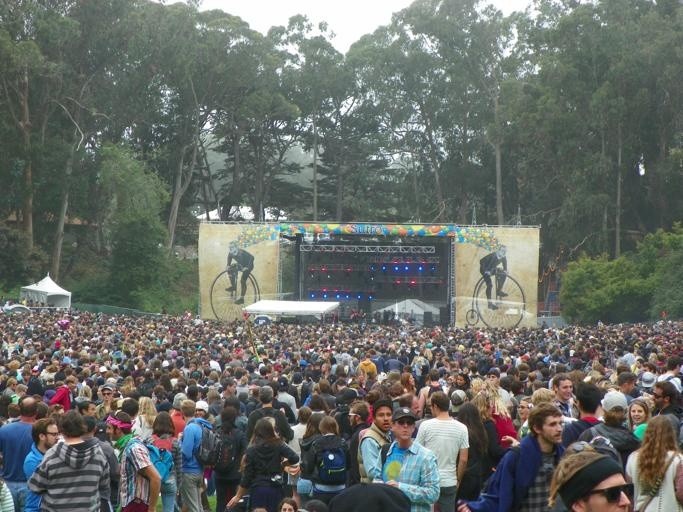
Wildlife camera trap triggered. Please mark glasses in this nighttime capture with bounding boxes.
[517,405,530,410]
[651,390,672,399]
[348,413,360,417]
[586,482,635,504]
[102,393,112,396]
[373,400,393,412]
[398,418,415,426]
[195,400,209,413]
[45,430,61,437]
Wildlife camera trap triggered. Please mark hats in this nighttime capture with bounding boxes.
[641,371,657,387]
[299,359,309,365]
[172,393,187,410]
[391,407,420,423]
[601,391,627,414]
[450,389,466,413]
[100,386,114,392]
[99,366,108,374]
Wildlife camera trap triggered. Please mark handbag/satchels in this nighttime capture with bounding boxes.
[590,426,624,473]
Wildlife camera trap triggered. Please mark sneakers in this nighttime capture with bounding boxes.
[226,286,237,291]
[235,299,244,305]
[488,304,498,310]
[496,291,508,298]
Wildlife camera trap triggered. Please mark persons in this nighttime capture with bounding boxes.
[355,397,390,482]
[297,304,360,494]
[150,412,184,512]
[249,503,266,512]
[501,395,531,451]
[225,419,300,510]
[120,308,297,430]
[457,403,488,501]
[22,410,113,512]
[361,325,527,406]
[528,321,618,405]
[278,495,299,511]
[414,391,472,512]
[303,497,327,511]
[454,400,567,512]
[624,412,683,512]
[548,449,630,512]
[373,406,441,512]
[361,307,417,326]
[476,390,509,462]
[179,400,209,512]
[281,497,282,500]
[8,395,33,511]
[619,319,683,410]
[479,244,514,310]
[563,380,625,446]
[223,237,255,306]
[104,409,163,510]
[0,295,120,417]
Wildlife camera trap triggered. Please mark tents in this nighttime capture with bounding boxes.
[372,298,442,316]
[240,298,341,326]
[19,270,71,312]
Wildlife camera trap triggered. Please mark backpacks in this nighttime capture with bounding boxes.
[218,434,238,475]
[319,446,345,484]
[200,430,216,464]
